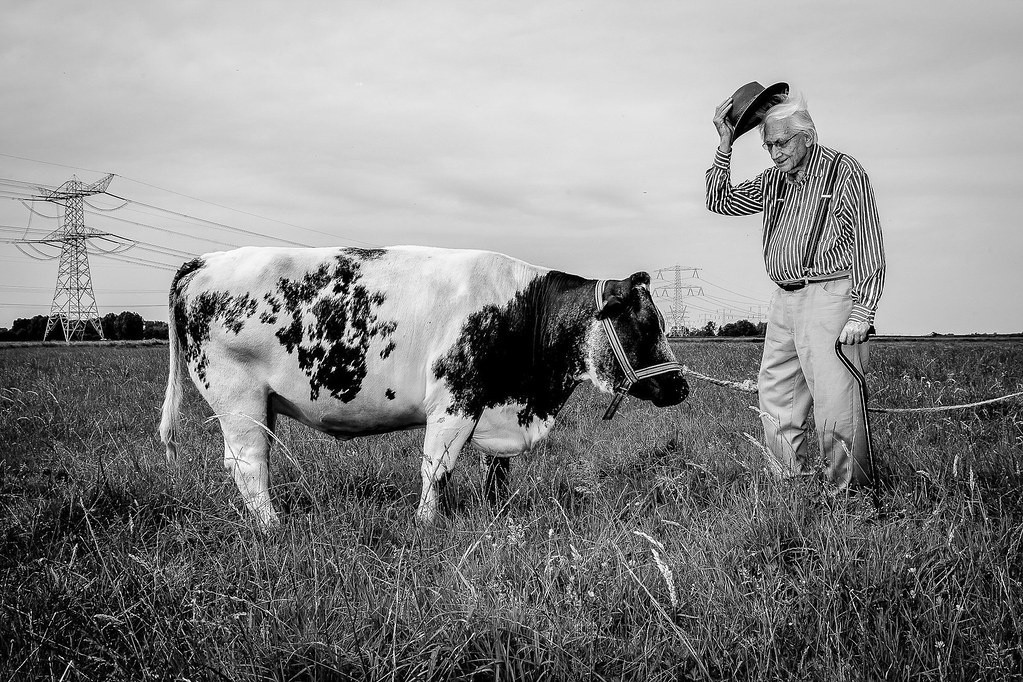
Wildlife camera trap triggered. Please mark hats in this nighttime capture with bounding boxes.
[723,80,789,146]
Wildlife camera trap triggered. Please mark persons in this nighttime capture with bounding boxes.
[705,81,886,510]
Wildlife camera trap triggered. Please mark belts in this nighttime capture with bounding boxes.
[775,274,849,291]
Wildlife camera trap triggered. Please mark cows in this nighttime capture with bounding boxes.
[160,244,691,530]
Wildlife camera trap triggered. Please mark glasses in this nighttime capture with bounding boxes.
[761,132,800,150]
